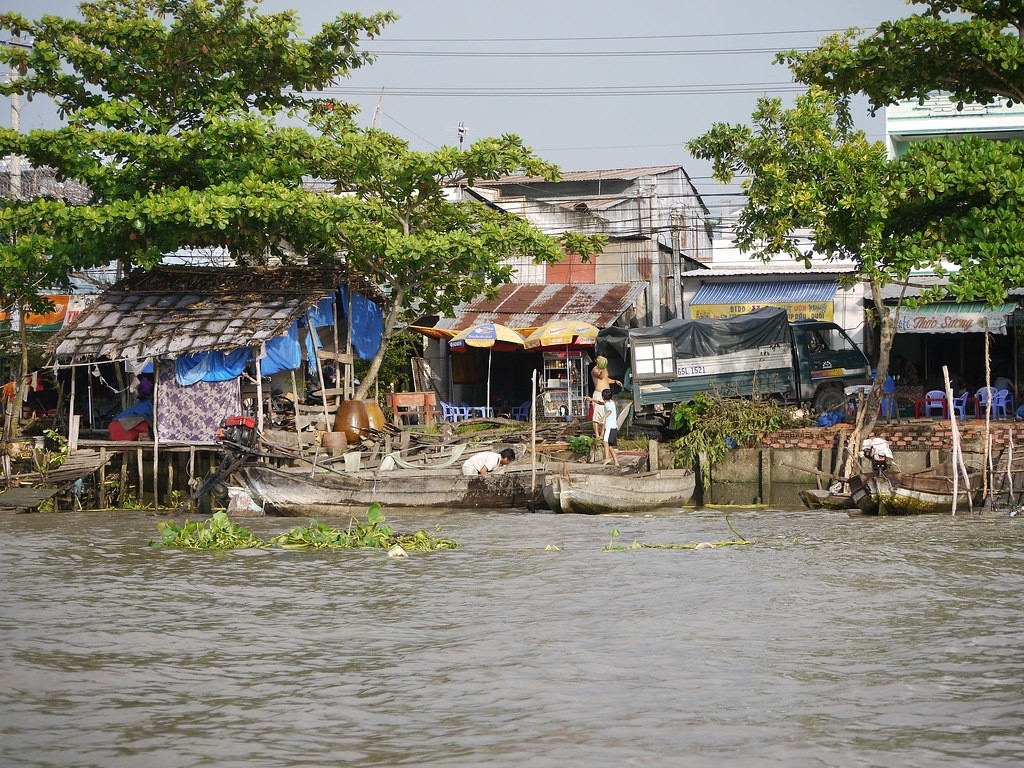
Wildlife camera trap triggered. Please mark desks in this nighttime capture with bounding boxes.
[916,398,948,419]
[967,397,982,420]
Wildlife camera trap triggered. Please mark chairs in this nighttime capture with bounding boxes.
[439,401,467,423]
[948,392,969,421]
[512,401,531,422]
[449,401,494,418]
[925,390,945,418]
[987,389,1008,420]
[974,387,998,420]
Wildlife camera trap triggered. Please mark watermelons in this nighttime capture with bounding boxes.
[598,357,608,370]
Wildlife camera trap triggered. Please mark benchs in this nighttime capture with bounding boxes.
[385,391,441,426]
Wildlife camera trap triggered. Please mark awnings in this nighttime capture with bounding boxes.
[689,281,841,322]
[884,302,1020,335]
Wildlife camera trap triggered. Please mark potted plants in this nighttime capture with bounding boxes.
[566,434,604,463]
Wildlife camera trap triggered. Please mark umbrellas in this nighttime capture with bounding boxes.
[447,323,523,417]
[523,319,599,420]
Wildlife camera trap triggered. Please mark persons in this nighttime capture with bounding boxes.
[462,448,516,476]
[587,389,620,467]
[994,368,1015,393]
[894,354,918,385]
[590,352,623,439]
[138,374,153,396]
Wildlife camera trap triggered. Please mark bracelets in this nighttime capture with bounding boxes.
[615,380,616,383]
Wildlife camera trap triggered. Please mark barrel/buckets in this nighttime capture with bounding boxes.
[872,369,895,417]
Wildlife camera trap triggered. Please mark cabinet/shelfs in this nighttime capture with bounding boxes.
[543,351,590,417]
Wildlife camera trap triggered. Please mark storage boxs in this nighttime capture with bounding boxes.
[548,379,561,387]
